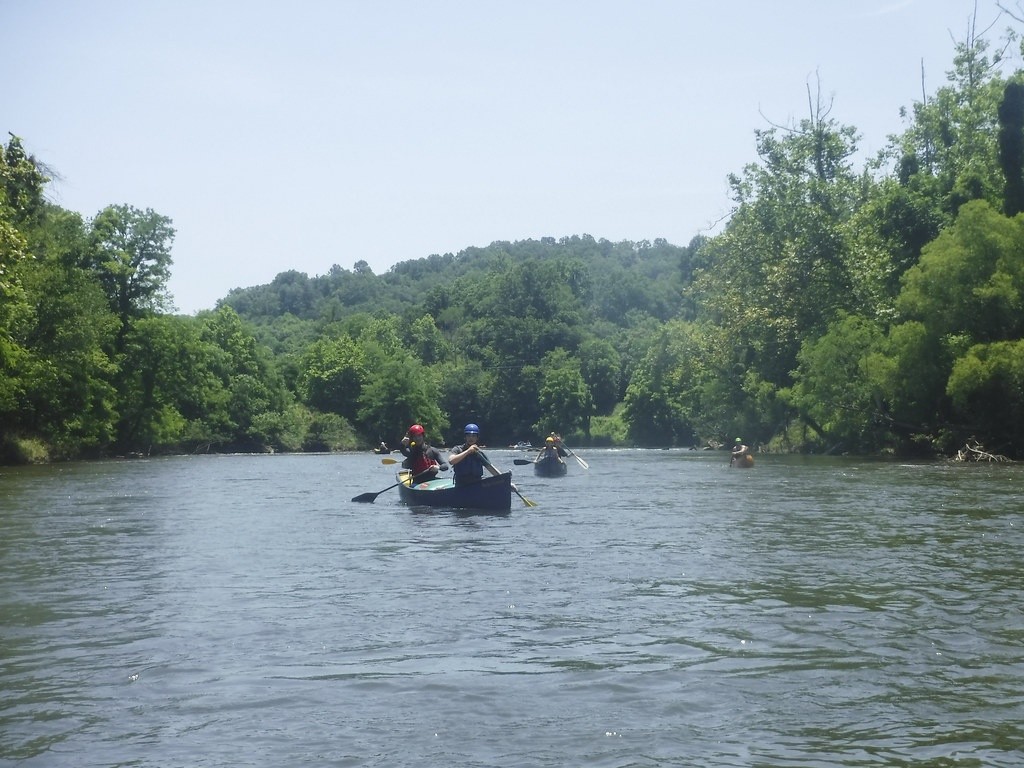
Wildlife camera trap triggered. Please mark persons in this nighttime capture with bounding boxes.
[534,434,575,464]
[399,424,449,488]
[380,442,388,450]
[730,437,751,461]
[449,424,518,492]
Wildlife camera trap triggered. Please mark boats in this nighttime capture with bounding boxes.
[396,467,512,513]
[735,454,754,468]
[534,454,568,478]
[374,448,390,454]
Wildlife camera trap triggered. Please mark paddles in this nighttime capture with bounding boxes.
[352,469,430,503]
[381,458,404,465]
[729,455,734,469]
[512,459,539,466]
[551,430,589,469]
[474,446,536,507]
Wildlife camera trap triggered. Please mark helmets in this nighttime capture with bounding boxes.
[735,437,741,443]
[553,437,560,442]
[464,423,479,434]
[410,442,416,448]
[381,442,384,445]
[410,424,424,435]
[546,436,553,442]
